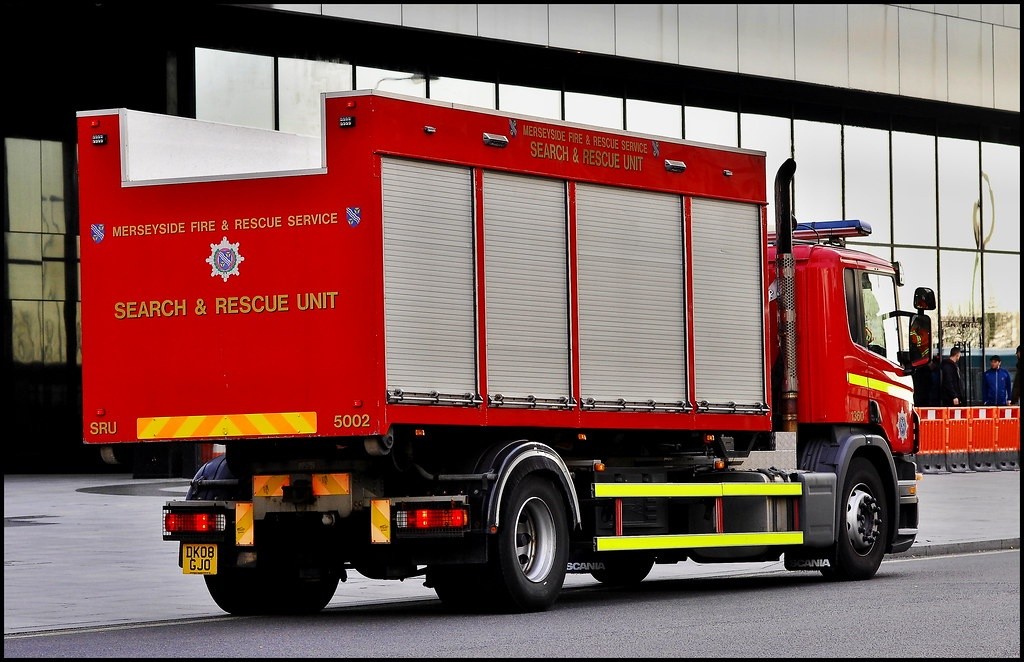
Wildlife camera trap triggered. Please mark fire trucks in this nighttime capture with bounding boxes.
[75,90,936,616]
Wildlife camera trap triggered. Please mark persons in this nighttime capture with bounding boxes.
[982,345,1020,406]
[915,297,929,309]
[910,317,930,366]
[940,347,962,407]
